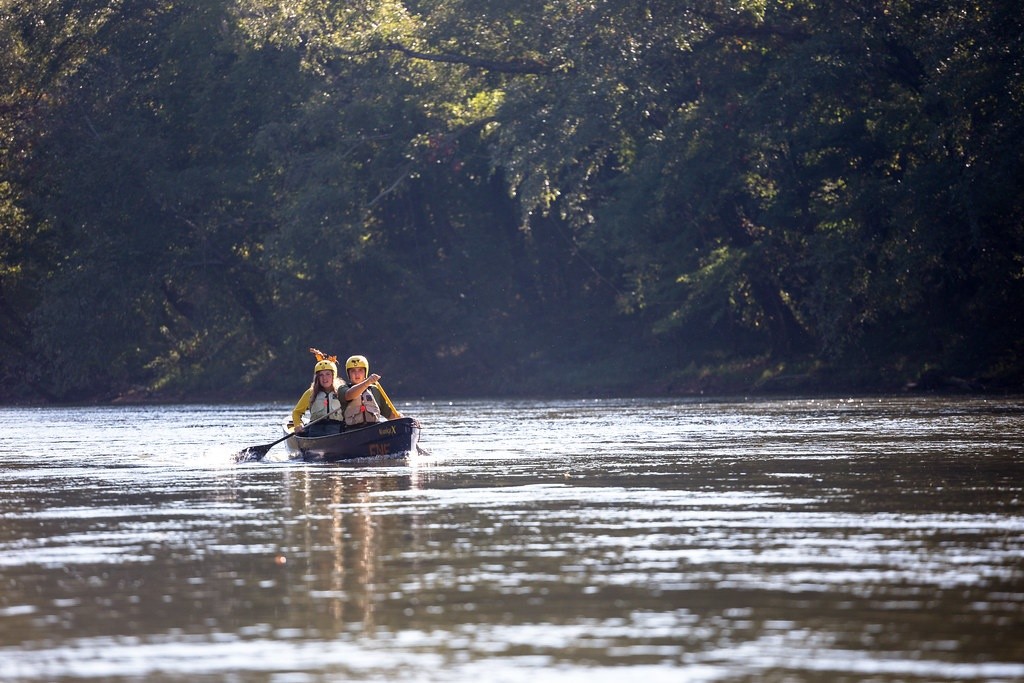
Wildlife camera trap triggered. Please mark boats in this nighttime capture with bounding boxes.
[280,414,421,465]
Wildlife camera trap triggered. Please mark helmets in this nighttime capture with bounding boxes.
[345,355,369,380]
[314,359,337,387]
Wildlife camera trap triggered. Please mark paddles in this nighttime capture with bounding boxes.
[234,406,341,463]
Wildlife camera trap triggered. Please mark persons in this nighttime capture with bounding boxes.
[337,355,404,435]
[292,360,343,438]
